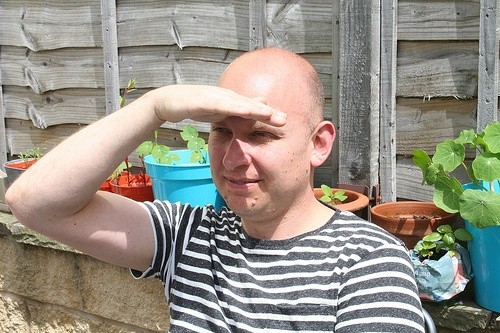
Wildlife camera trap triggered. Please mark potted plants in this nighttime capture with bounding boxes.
[4,77,500,313]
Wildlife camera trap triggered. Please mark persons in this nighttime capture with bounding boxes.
[5,48,425,333]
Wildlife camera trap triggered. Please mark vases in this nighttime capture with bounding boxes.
[371,201,456,250]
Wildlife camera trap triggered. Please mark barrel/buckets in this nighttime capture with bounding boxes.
[459,181,500,313]
[144,149,228,213]
